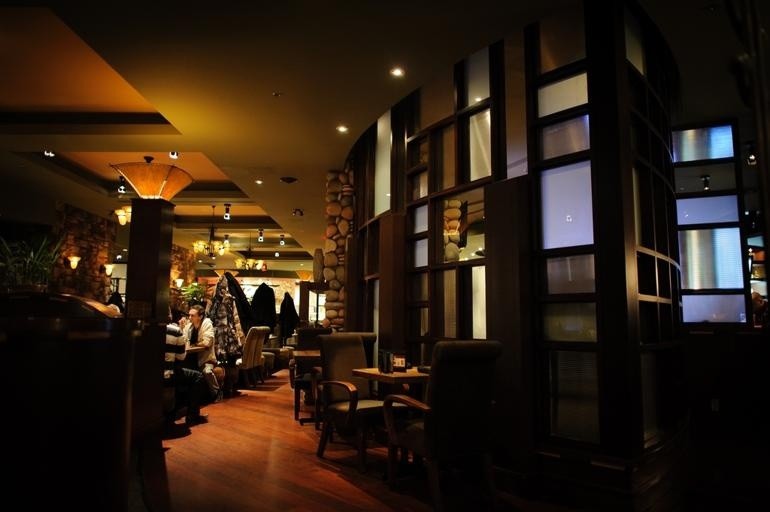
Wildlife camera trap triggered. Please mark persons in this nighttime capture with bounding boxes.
[166,305,209,425]
[177,304,223,404]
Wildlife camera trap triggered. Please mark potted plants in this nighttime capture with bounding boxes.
[0,236,62,294]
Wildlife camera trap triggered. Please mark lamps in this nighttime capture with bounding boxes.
[66,152,285,277]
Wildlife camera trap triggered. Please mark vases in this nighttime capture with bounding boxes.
[314,250,322,282]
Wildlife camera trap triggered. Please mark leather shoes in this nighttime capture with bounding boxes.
[186,415,208,424]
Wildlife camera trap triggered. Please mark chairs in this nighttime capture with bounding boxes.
[164,327,294,436]
[289,327,510,506]
